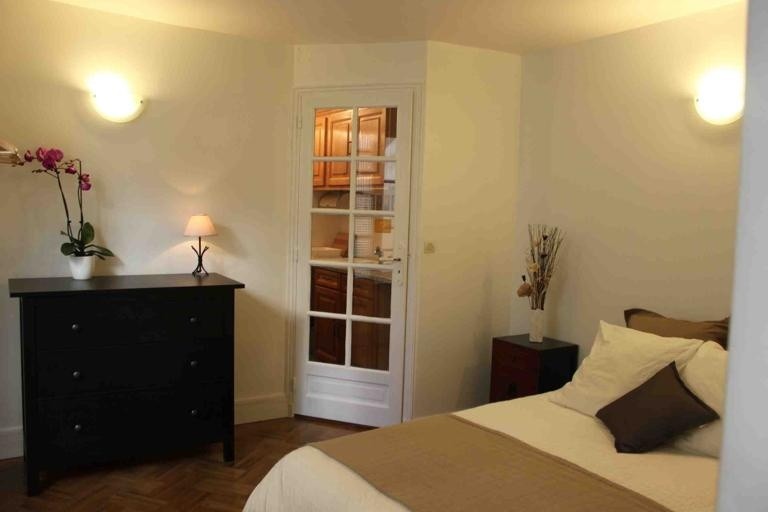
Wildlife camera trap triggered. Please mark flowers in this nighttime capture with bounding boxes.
[13,146,115,263]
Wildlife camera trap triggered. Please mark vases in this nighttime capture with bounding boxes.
[67,254,97,280]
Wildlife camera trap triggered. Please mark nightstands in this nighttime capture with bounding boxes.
[490,332,578,404]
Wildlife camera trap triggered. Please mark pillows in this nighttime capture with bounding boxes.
[545,307,729,462]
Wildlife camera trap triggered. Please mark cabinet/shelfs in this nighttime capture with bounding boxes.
[313,269,391,369]
[327,106,398,187]
[315,115,326,187]
[7,271,245,499]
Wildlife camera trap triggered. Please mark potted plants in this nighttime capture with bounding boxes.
[516,222,565,345]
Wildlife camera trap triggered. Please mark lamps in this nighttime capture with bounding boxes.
[184,214,219,277]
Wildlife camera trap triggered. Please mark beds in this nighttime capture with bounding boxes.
[265,391,724,512]
